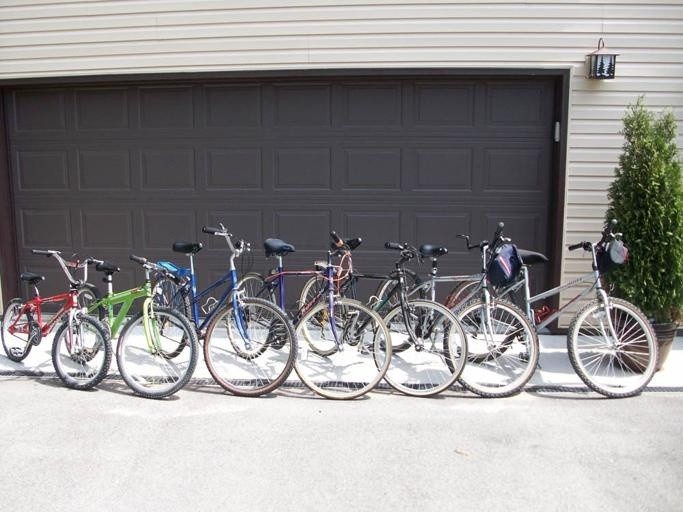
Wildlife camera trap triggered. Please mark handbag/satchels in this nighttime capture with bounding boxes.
[592,237,628,273]
[489,244,524,286]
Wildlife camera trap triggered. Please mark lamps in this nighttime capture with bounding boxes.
[583,39,621,79]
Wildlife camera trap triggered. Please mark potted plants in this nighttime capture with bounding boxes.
[596,95,682,372]
